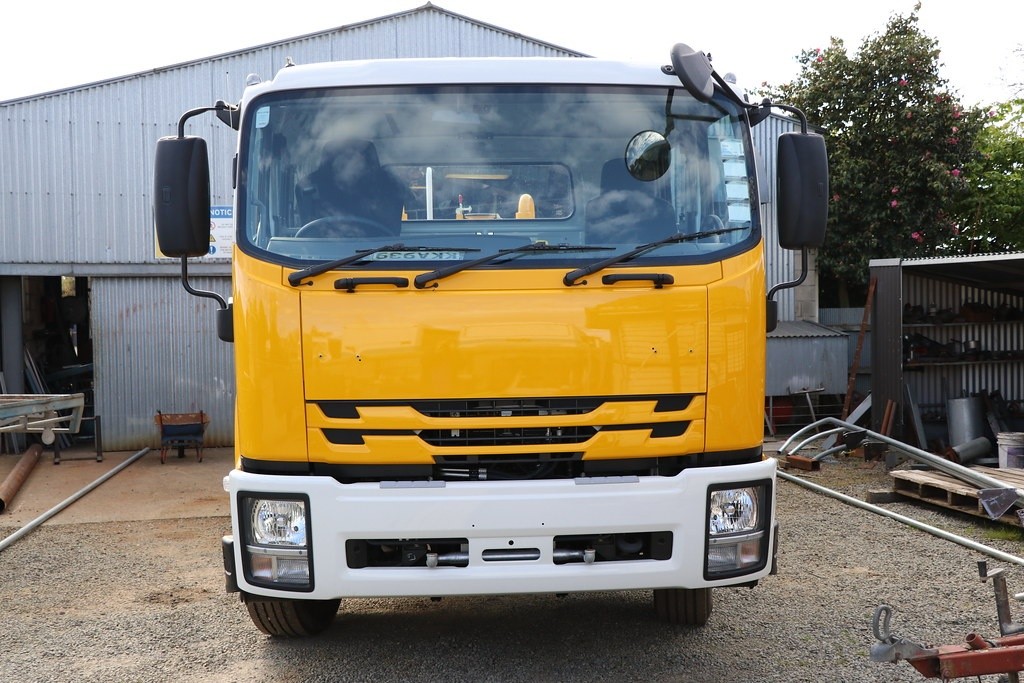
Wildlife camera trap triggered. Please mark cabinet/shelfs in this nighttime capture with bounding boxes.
[903,317,1024,422]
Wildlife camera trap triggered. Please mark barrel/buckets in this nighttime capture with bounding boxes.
[947,396,986,448]
[996,431,1024,469]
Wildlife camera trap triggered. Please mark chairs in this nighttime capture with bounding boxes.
[296,138,417,239]
[586,159,679,246]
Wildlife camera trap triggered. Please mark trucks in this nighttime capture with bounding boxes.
[154,40,830,640]
